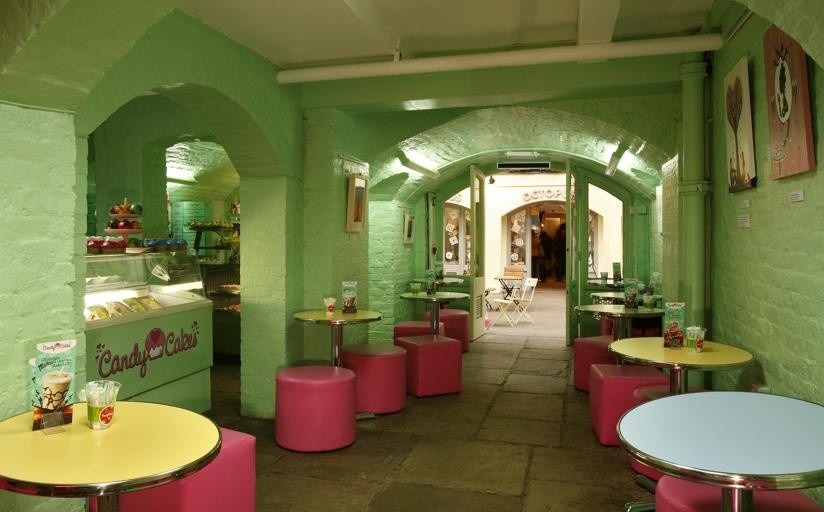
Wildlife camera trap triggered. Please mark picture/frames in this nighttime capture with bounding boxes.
[346,173,368,232]
[763,22,816,181]
[724,57,758,194]
[404,214,414,244]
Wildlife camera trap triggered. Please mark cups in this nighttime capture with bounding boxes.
[410,282,421,294]
[628,291,636,303]
[85,380,121,430]
[642,295,657,310]
[42,372,71,408]
[344,293,355,306]
[685,326,707,352]
[324,296,336,314]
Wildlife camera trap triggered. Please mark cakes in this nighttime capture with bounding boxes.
[86,235,127,254]
[144,236,188,251]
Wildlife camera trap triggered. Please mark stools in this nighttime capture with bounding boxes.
[600,316,659,339]
[426,309,470,353]
[572,336,616,392]
[395,320,444,337]
[396,334,461,397]
[590,365,670,446]
[343,344,407,414]
[276,365,356,452]
[90,428,255,512]
[655,474,759,512]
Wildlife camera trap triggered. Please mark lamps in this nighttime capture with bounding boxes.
[602,143,636,176]
[392,149,439,180]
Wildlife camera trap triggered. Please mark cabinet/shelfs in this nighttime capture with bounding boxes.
[194,223,242,355]
[85,250,212,414]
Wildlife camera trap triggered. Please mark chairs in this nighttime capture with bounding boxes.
[507,278,538,325]
[491,287,515,328]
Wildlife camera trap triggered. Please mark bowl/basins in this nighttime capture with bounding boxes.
[91,276,108,284]
[109,275,122,282]
